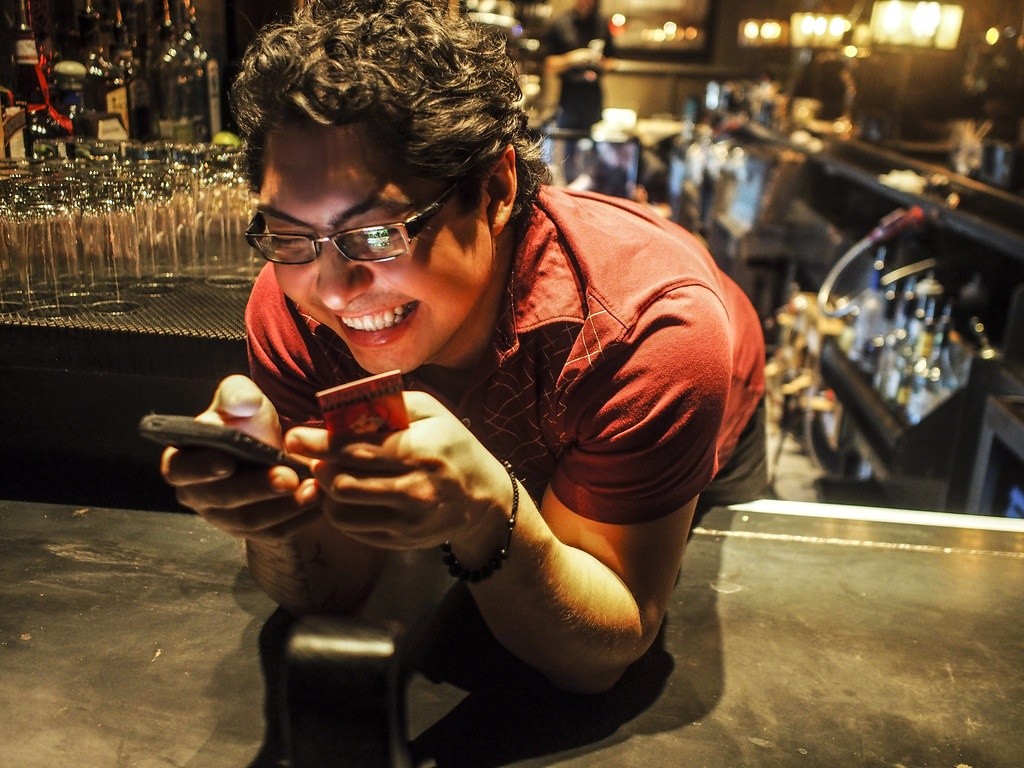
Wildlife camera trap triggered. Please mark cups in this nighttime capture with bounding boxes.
[0,142,270,318]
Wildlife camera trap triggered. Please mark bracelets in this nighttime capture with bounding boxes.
[441,458,519,583]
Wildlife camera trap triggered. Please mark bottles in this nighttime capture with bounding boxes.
[0,0,224,155]
[850,245,973,429]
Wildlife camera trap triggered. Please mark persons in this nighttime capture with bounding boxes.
[542,0,617,183]
[160,0,766,694]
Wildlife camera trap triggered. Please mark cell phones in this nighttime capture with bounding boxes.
[140,415,320,485]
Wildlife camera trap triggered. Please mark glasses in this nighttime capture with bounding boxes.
[243,185,454,263]
